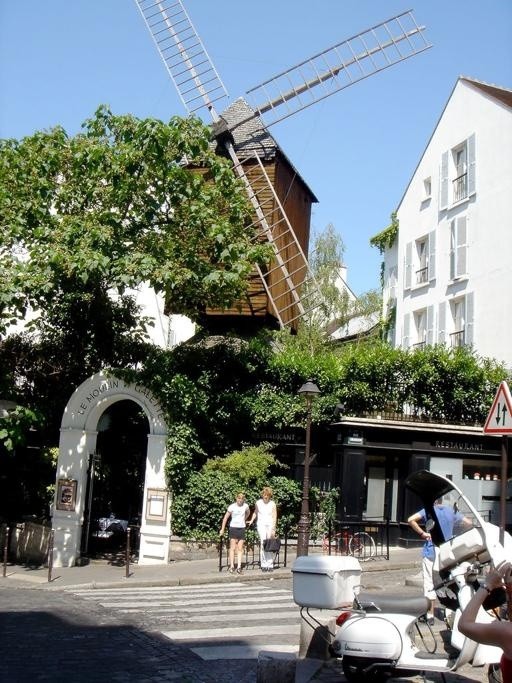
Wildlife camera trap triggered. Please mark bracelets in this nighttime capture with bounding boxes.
[419,531,424,536]
[478,582,492,594]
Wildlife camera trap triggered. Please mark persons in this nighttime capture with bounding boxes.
[218,490,250,575]
[244,485,279,574]
[406,496,474,626]
[457,558,511,682]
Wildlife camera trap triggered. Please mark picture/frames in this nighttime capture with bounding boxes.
[146,489,169,522]
[57,479,77,511]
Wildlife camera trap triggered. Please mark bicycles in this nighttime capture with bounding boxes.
[323,526,377,561]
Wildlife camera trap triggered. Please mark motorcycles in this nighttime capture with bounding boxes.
[291,470,512,681]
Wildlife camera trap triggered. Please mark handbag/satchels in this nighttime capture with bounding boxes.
[263,532,280,552]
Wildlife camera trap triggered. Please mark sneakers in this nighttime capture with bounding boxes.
[261,567,267,571]
[419,613,434,624]
[268,567,274,572]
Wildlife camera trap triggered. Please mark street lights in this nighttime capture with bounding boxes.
[296,378,321,557]
